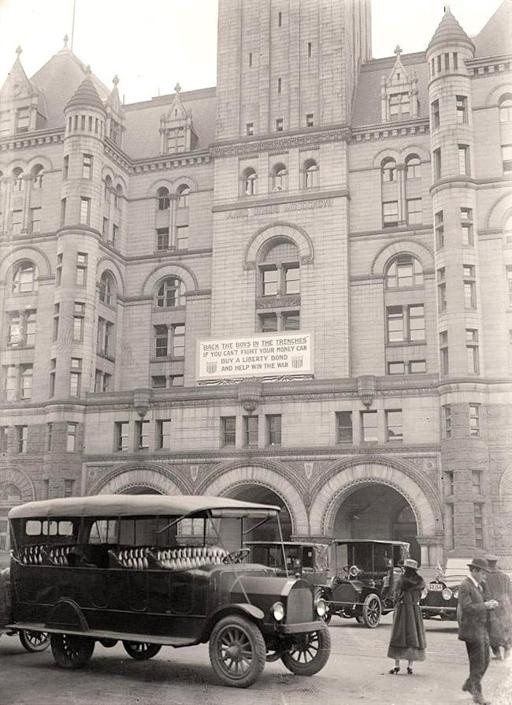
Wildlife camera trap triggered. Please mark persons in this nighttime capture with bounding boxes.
[461,556,498,705]
[387,557,427,676]
[484,557,512,662]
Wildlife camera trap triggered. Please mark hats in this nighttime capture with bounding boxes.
[401,559,420,570]
[467,559,498,574]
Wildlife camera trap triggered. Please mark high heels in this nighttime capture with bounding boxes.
[391,667,412,675]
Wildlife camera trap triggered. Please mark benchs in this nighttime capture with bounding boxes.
[20,543,229,572]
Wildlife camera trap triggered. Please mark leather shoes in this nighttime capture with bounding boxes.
[463,679,491,705]
